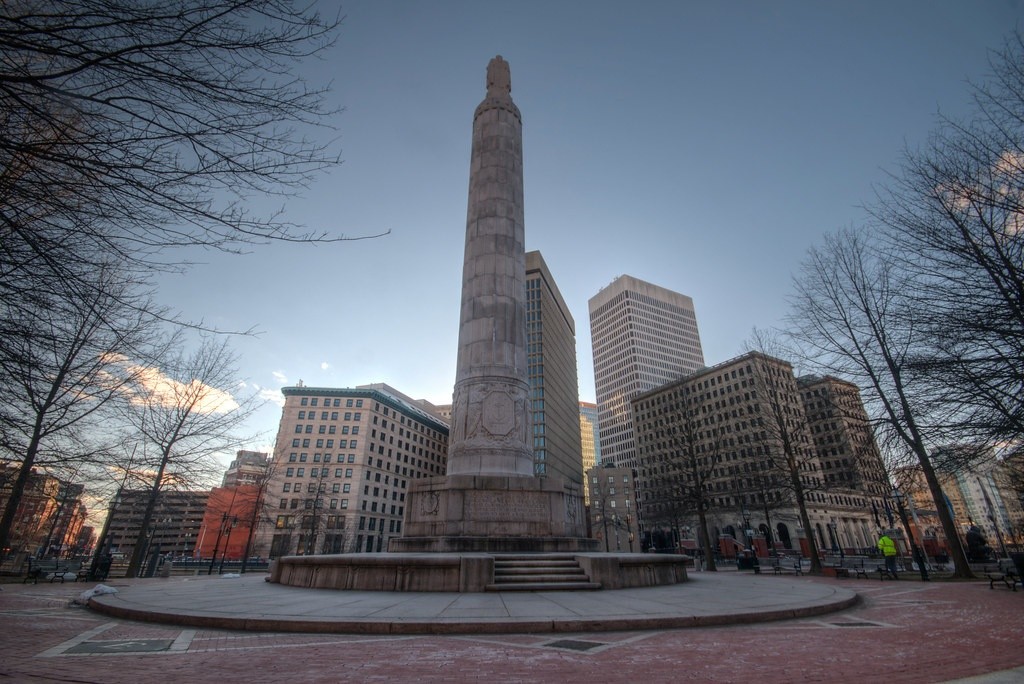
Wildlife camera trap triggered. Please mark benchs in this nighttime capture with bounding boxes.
[984,558,1022,592]
[23,559,69,584]
[833,558,894,581]
[75,561,105,583]
[774,556,804,577]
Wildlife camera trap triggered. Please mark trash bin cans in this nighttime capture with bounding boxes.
[89,554,114,581]
[693,558,702,571]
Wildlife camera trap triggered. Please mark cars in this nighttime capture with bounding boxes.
[10,555,35,561]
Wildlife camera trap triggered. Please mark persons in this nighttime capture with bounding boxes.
[876,530,899,580]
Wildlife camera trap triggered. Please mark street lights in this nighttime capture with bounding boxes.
[139,529,156,577]
[830,516,845,558]
[889,483,929,581]
[745,513,759,573]
